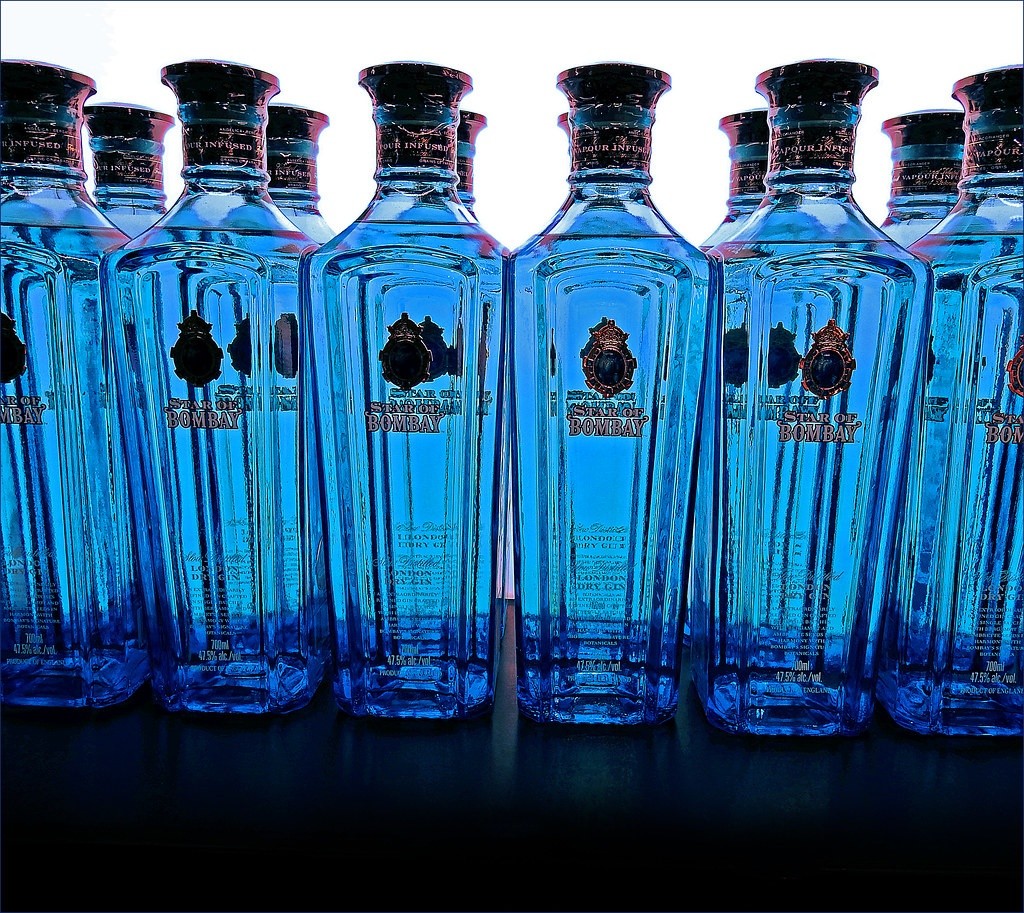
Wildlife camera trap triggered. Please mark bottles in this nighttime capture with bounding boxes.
[264,104,338,242]
[879,66,1023,739]
[500,62,721,727]
[0,58,150,710]
[80,97,176,239]
[696,107,771,252]
[297,60,510,717]
[154,58,329,714]
[708,60,939,738]
[874,106,963,248]
